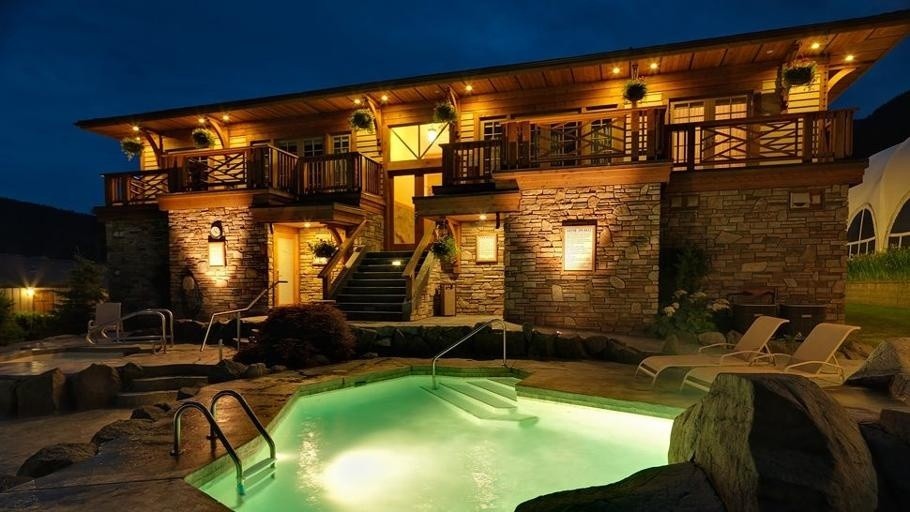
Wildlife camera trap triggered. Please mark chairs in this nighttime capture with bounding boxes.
[633,315,862,394]
[86,300,122,344]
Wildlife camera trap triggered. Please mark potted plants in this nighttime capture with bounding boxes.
[432,238,456,262]
[623,79,648,103]
[433,101,460,123]
[309,238,335,257]
[781,58,817,93]
[191,129,215,148]
[121,137,144,161]
[349,108,377,134]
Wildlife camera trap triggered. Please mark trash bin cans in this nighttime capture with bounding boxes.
[137,305,161,335]
[733,304,776,335]
[780,304,827,342]
[441,283,455,316]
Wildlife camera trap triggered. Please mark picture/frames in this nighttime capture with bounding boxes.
[561,226,596,274]
[207,241,226,268]
[475,232,498,263]
